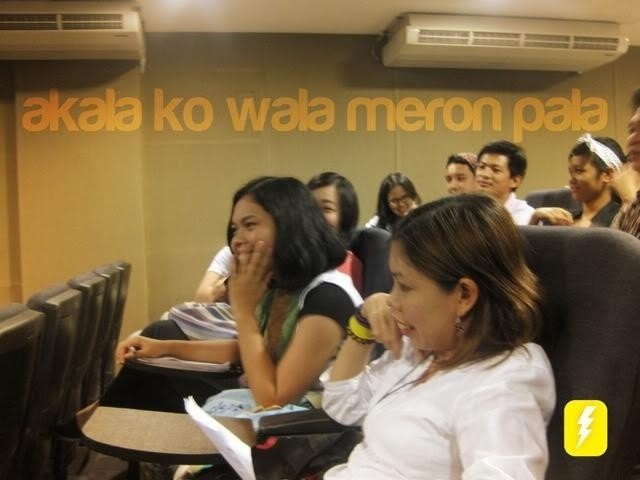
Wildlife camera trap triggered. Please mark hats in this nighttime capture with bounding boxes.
[460,153,477,170]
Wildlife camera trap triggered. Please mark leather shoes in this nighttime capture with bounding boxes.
[52,415,84,441]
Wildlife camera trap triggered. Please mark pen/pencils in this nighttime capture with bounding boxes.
[128,344,137,354]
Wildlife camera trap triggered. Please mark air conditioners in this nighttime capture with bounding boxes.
[0,0,144,62]
[380,12,628,70]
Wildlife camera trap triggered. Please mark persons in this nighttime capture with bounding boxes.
[44,88,638,479]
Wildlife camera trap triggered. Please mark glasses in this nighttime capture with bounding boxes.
[389,195,411,205]
